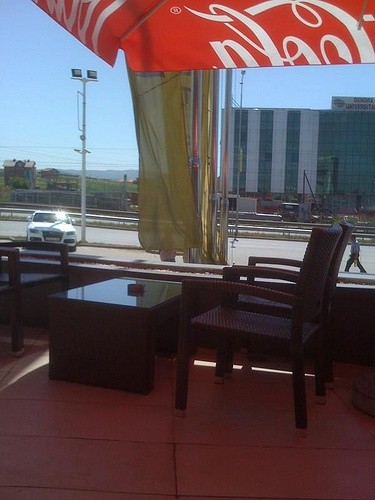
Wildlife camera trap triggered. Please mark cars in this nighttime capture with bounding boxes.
[26,210,78,252]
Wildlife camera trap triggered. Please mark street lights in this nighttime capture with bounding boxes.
[71,67,98,244]
[231,69,248,235]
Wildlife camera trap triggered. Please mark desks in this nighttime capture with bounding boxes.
[48,276,182,395]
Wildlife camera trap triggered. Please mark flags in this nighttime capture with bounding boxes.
[128,70,228,265]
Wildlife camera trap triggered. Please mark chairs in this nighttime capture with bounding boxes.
[0,242,70,358]
[173,221,354,429]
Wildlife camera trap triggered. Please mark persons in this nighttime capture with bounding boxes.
[345,235,367,273]
